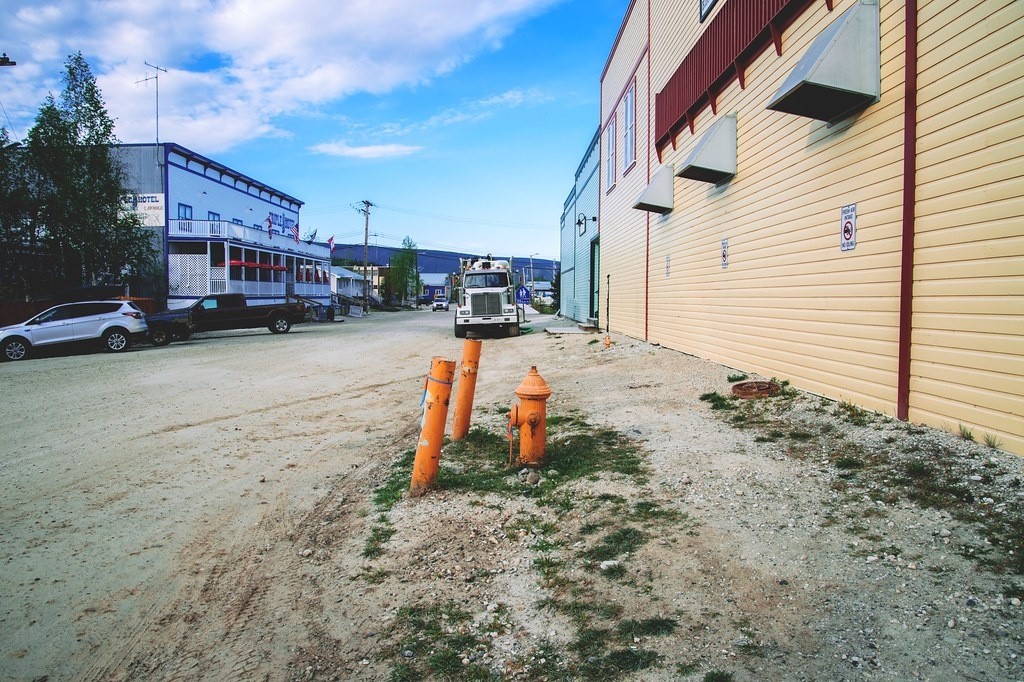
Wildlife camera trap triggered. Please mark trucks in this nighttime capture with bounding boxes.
[453,255,523,338]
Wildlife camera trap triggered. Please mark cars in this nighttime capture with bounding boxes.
[432,298,450,311]
[409,295,430,306]
[0,300,149,362]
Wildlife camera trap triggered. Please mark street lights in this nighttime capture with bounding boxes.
[530,252,540,299]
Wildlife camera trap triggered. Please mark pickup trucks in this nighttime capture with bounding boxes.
[146,293,308,346]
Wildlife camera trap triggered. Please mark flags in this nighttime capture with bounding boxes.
[266,217,273,239]
[291,223,300,243]
[307,231,316,245]
[328,237,335,252]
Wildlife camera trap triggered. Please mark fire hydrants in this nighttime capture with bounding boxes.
[507,365,554,466]
